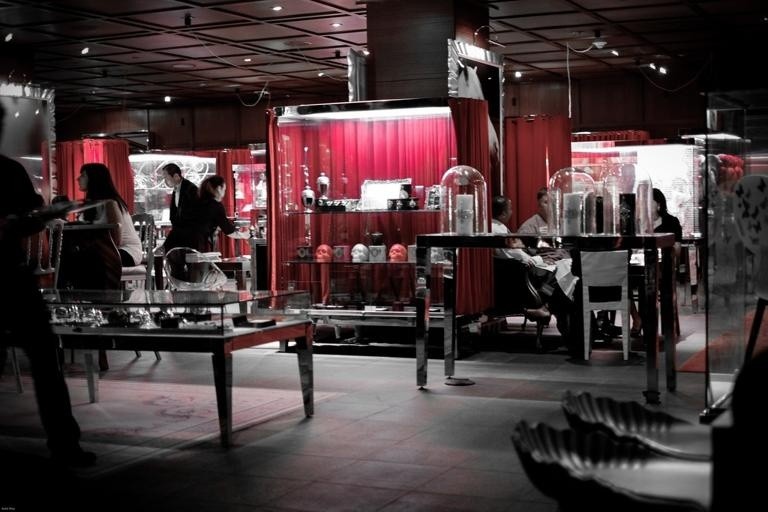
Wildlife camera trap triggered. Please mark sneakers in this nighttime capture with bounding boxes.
[630,314,644,338]
[590,310,623,342]
[47,439,97,473]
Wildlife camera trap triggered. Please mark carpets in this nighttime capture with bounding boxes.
[1,373,345,488]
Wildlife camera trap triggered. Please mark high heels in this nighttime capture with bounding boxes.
[525,306,552,325]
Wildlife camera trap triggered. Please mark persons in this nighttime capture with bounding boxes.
[516,188,551,236]
[160,175,237,254]
[0,100,98,470]
[491,192,568,346]
[160,163,198,225]
[74,162,143,265]
[629,187,682,335]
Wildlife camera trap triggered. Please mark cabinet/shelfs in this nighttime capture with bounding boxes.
[14,96,491,448]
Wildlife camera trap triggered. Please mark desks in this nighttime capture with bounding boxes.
[409,234,701,409]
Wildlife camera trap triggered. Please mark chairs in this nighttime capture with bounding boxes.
[491,248,685,361]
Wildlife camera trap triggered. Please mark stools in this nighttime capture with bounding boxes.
[511,389,711,512]
